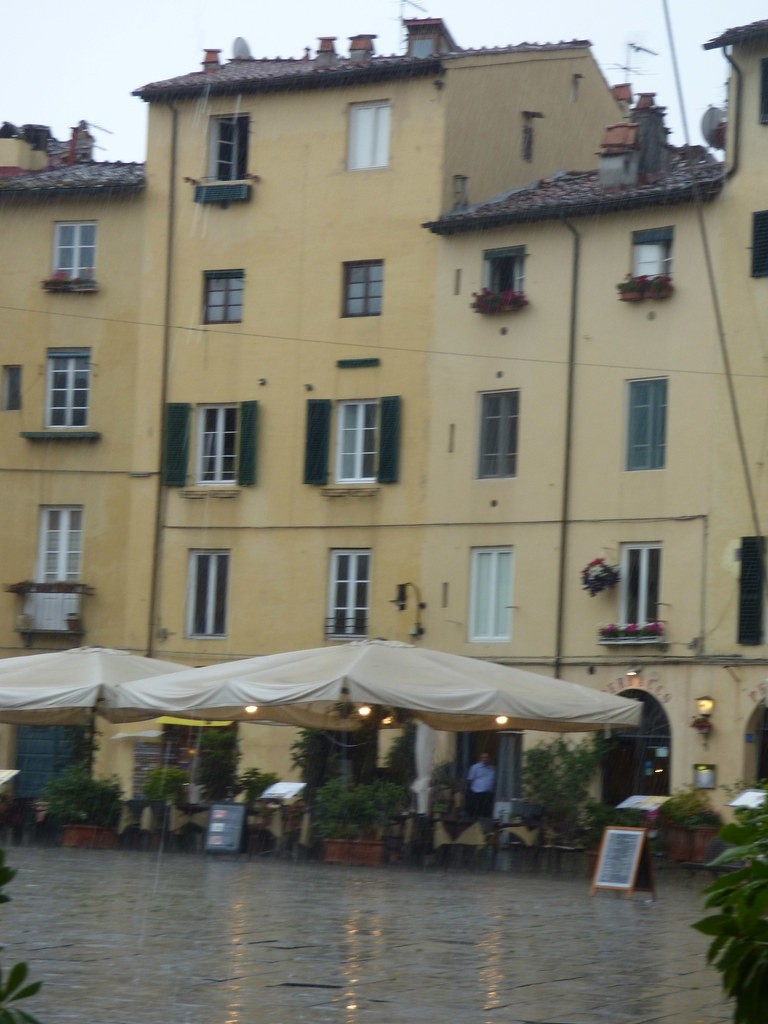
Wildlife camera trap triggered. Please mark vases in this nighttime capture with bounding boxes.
[622,289,669,299]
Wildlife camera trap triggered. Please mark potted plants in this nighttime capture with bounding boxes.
[315,776,419,869]
[38,767,124,850]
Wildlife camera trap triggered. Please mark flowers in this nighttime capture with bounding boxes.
[470,286,526,316]
[44,265,99,286]
[617,271,674,292]
[599,621,662,638]
[579,557,619,599]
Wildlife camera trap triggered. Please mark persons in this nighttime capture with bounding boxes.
[464,751,498,857]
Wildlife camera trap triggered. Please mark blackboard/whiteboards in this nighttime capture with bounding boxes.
[591,825,657,892]
[201,802,248,855]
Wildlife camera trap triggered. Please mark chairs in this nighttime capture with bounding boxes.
[121,799,588,880]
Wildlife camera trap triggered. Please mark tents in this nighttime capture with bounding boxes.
[109,638,643,863]
[0,644,213,786]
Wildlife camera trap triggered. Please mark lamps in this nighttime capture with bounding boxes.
[390,581,426,636]
[693,695,718,742]
